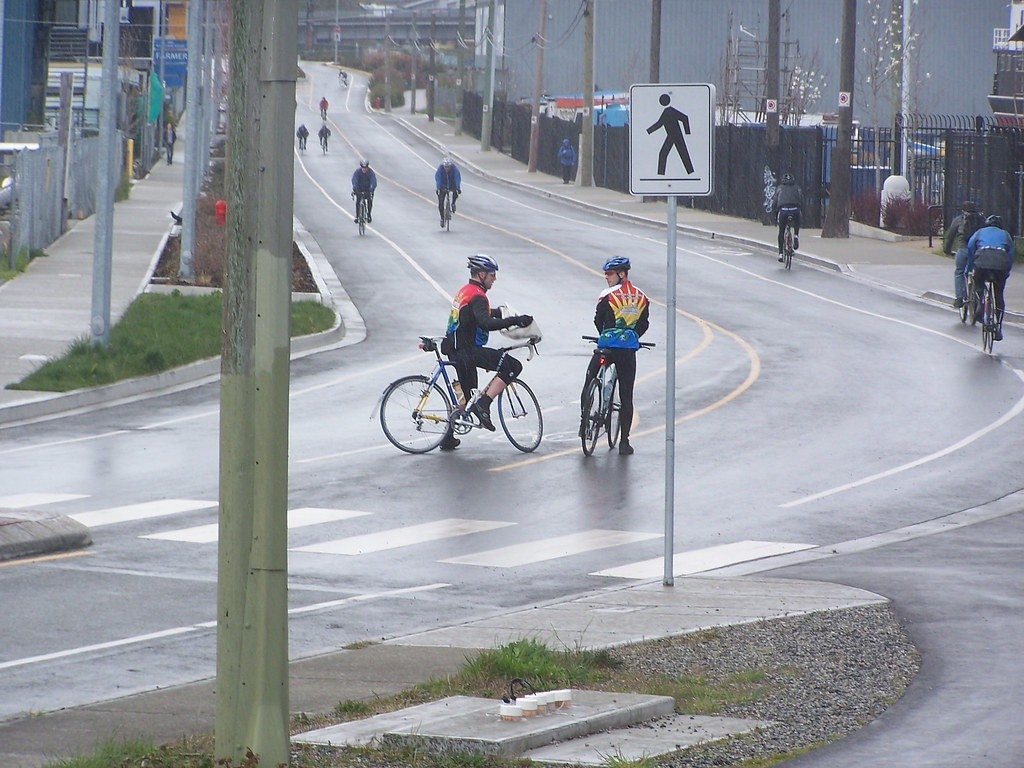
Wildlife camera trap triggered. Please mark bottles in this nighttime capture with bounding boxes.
[601,380,614,402]
[452,378,466,406]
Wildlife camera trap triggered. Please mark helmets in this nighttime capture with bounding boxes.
[563,139,569,145]
[984,214,1004,229]
[603,257,630,270]
[467,255,499,272]
[443,159,452,166]
[360,160,369,168]
[962,200,978,212]
[783,174,794,184]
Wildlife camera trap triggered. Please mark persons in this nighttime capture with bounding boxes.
[439,254,534,449]
[967,214,1014,340]
[578,255,650,454]
[318,125,331,151]
[352,159,377,223]
[320,97,328,121]
[943,201,986,307]
[339,69,347,86]
[772,174,802,262]
[297,124,309,150]
[163,123,177,165]
[435,158,461,227]
[559,139,576,184]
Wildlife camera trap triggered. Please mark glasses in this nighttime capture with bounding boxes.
[490,273,495,277]
[604,271,615,276]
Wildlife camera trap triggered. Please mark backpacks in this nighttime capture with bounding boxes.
[965,214,985,242]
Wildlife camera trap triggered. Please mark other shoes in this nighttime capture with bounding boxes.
[451,203,457,212]
[367,213,372,223]
[778,253,783,262]
[440,219,444,228]
[354,218,359,224]
[793,236,799,249]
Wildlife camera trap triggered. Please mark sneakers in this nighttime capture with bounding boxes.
[619,441,635,455]
[440,435,461,448]
[471,401,496,431]
[953,298,1003,341]
[578,424,592,436]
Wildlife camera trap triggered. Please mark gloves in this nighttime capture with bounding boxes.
[515,314,534,328]
[493,308,502,319]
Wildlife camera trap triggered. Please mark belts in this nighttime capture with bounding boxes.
[781,207,798,211]
